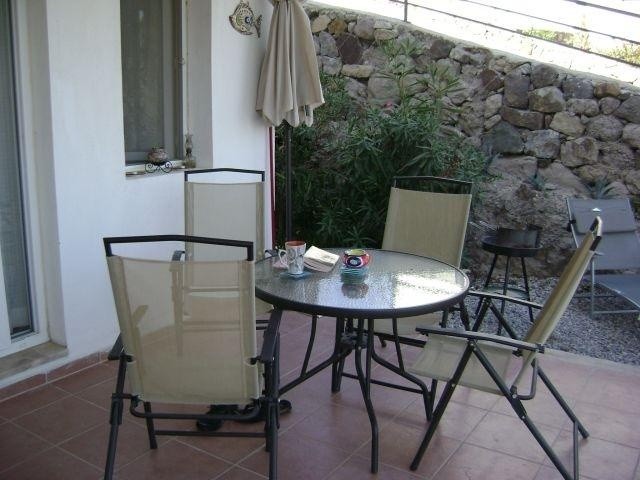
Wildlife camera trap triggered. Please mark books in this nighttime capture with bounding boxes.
[273,246,340,272]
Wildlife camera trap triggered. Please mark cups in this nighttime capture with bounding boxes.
[278,240,307,276]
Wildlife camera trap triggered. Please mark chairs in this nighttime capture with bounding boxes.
[410,215,603,479]
[565,197,640,317]
[169,167,281,360]
[104,235,283,480]
[333,175,475,422]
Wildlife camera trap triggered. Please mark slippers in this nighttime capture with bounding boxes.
[196,399,292,431]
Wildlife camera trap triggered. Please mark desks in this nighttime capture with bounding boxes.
[255,247,470,474]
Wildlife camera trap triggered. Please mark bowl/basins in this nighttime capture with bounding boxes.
[343,249,370,269]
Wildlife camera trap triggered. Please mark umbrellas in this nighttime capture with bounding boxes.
[255,0,325,240]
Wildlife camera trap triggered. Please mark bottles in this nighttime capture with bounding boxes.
[148,147,168,164]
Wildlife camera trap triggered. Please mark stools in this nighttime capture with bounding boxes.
[474,230,541,336]
